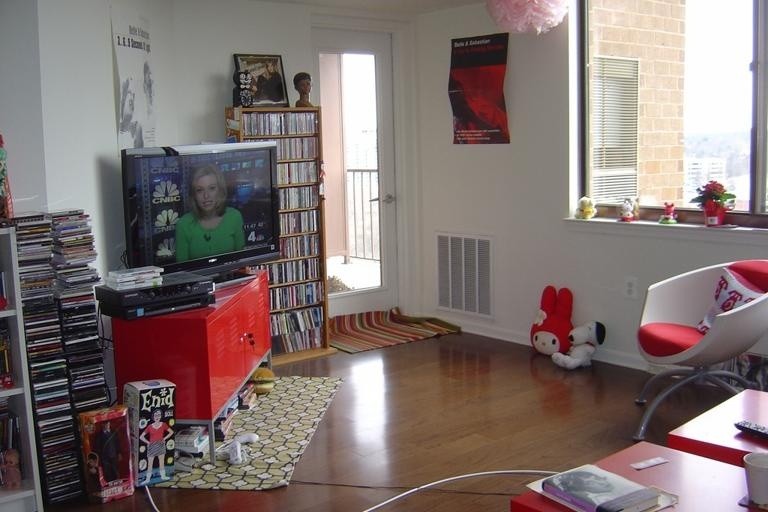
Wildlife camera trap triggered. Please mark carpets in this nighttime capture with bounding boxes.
[149,376,346,492]
[328,306,459,354]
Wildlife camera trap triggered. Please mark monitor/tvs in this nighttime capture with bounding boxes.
[121,140,281,290]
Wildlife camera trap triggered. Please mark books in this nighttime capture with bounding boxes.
[269,282,323,311]
[243,137,318,161]
[1,210,111,504]
[280,233,320,259]
[175,426,209,453]
[242,112,317,136]
[251,258,320,285]
[214,396,239,440]
[270,305,324,337]
[278,326,325,354]
[542,463,660,511]
[278,185,318,211]
[237,383,259,411]
[106,265,164,292]
[276,162,318,185]
[280,209,318,235]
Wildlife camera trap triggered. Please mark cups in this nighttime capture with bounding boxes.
[743,452,767,504]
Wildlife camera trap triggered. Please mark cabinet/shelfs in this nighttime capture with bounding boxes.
[112,269,272,464]
[223,106,338,368]
[0,227,45,512]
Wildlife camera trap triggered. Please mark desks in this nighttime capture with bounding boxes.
[667,388,768,468]
[511,441,768,512]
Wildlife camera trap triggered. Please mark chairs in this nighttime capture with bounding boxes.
[632,258,768,442]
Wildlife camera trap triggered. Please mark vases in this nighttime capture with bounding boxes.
[703,208,726,227]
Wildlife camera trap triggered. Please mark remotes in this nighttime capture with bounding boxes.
[735,420,768,438]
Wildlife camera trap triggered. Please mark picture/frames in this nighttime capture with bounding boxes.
[234,53,290,108]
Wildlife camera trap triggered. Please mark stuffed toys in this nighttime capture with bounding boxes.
[530,285,573,356]
[550,320,606,370]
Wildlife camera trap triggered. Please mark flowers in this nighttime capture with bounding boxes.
[689,180,736,208]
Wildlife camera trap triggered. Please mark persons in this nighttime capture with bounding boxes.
[139,410,174,486]
[256,59,284,102]
[293,73,316,107]
[94,420,122,481]
[174,164,246,264]
